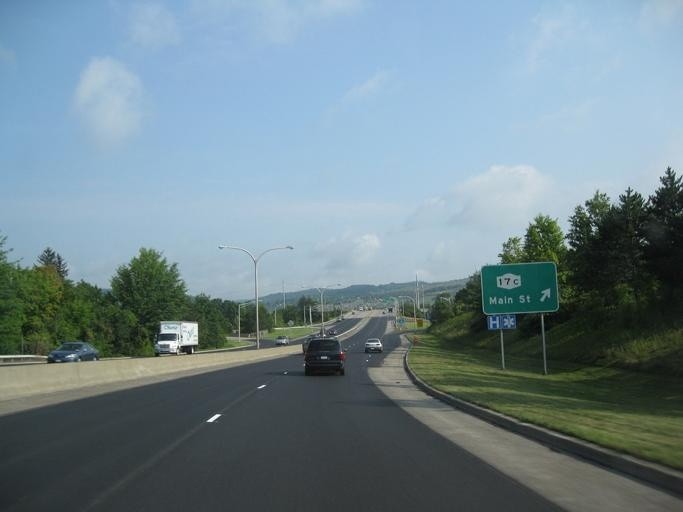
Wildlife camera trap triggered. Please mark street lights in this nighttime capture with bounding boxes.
[238,299,264,344]
[301,283,341,328]
[218,246,294,349]
[391,296,416,332]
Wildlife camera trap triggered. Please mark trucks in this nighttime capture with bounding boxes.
[153,320,199,357]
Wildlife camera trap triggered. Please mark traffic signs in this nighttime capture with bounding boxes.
[480,261,560,315]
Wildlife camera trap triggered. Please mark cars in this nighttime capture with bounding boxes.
[314,329,336,338]
[47,342,99,363]
[304,336,346,376]
[365,338,384,353]
[276,336,289,345]
